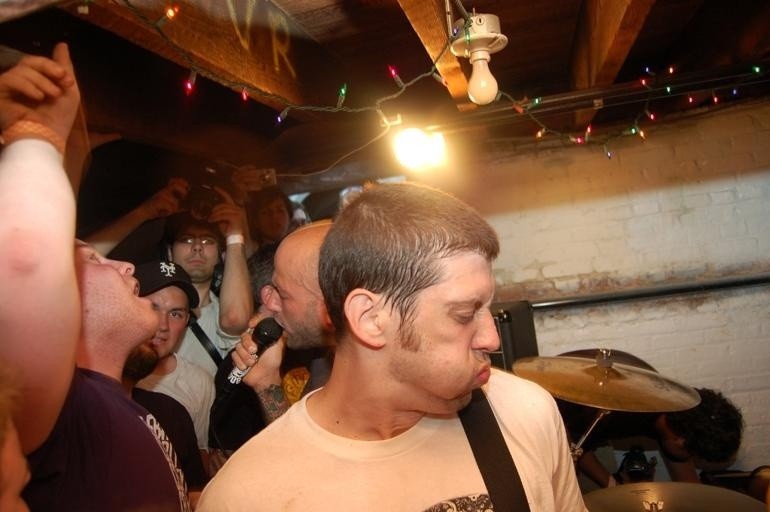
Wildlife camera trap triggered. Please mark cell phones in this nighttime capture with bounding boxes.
[238,168,277,192]
[173,177,212,201]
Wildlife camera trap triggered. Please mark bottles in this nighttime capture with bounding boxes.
[132,259,200,311]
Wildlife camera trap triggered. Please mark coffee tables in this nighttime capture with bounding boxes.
[512,349,701,412]
[582,482,768,512]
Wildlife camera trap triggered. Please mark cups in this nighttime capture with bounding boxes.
[175,233,219,246]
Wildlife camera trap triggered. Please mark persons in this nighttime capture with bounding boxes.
[553,349,743,495]
[193,193,590,512]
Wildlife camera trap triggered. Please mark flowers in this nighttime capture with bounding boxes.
[227,317,284,385]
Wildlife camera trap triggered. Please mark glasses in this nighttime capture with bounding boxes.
[450,13,508,106]
